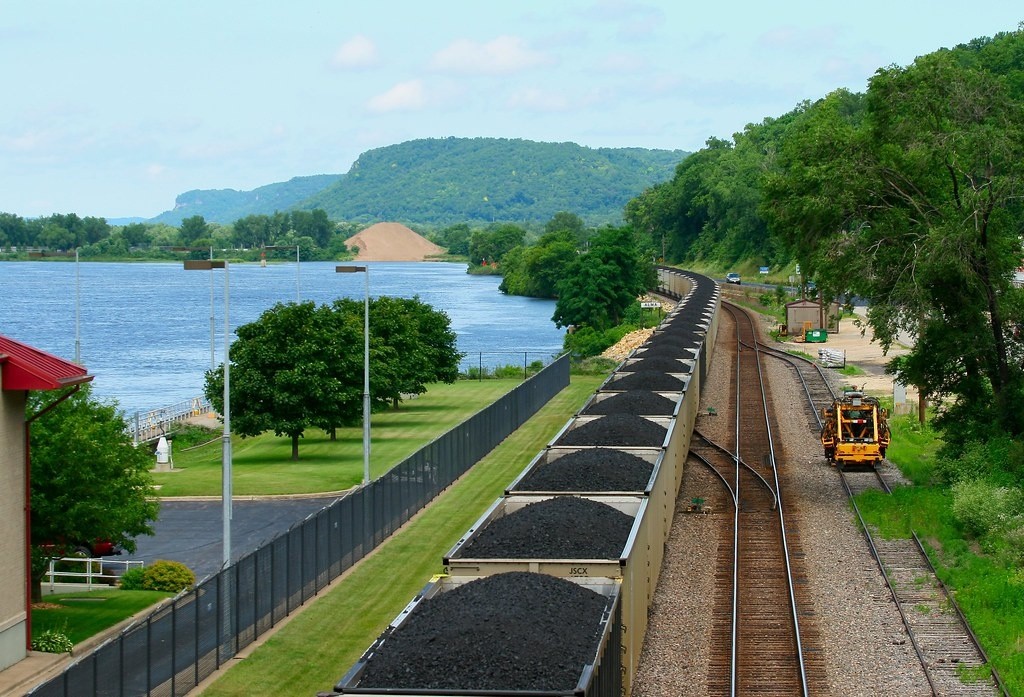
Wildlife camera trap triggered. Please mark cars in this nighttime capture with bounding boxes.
[726,272,742,285]
[33,531,123,559]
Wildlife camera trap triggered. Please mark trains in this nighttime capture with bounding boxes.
[317,265,721,697]
[821,389,891,470]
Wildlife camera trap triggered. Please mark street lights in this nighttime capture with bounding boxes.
[172,247,215,374]
[180,260,232,665]
[266,245,300,307]
[336,266,371,546]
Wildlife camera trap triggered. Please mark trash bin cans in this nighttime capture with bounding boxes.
[806,329,827,342]
